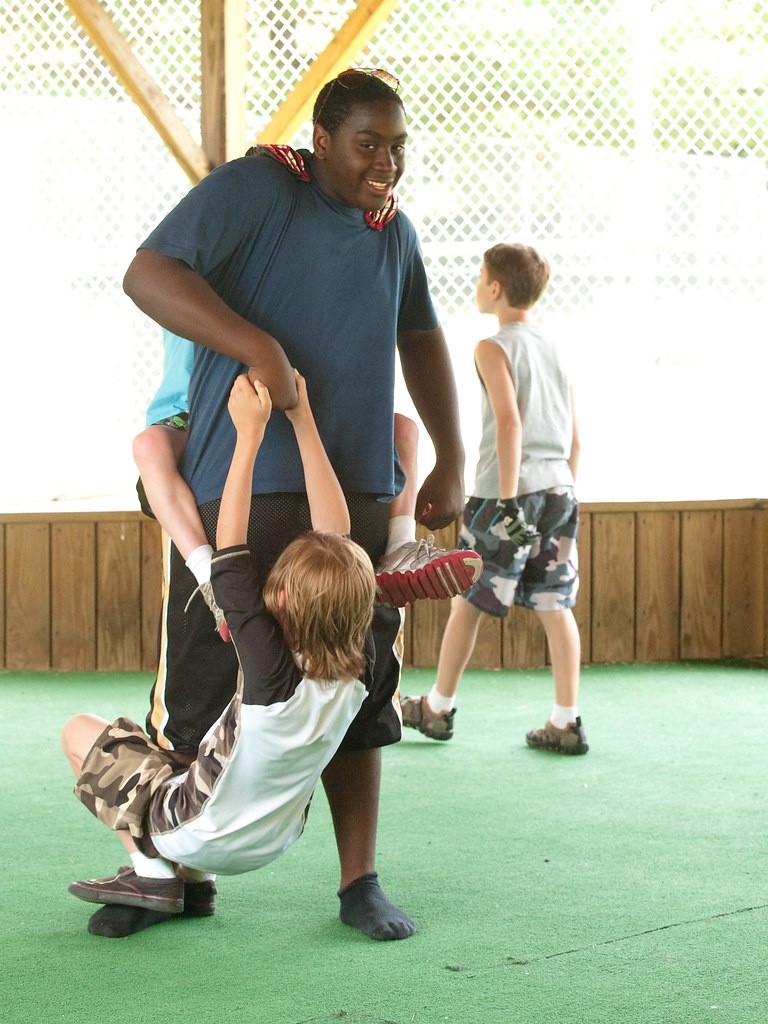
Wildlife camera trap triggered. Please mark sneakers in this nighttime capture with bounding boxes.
[184,879,217,915]
[400,695,457,740]
[375,533,484,609]
[68,865,184,913]
[526,716,589,755]
[183,581,230,642]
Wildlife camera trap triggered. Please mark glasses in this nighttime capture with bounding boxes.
[313,67,400,125]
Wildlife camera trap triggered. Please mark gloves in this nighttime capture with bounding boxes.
[499,498,541,547]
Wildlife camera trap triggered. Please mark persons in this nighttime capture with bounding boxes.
[59,368,378,915]
[397,242,588,754]
[130,326,481,645]
[86,72,468,942]
